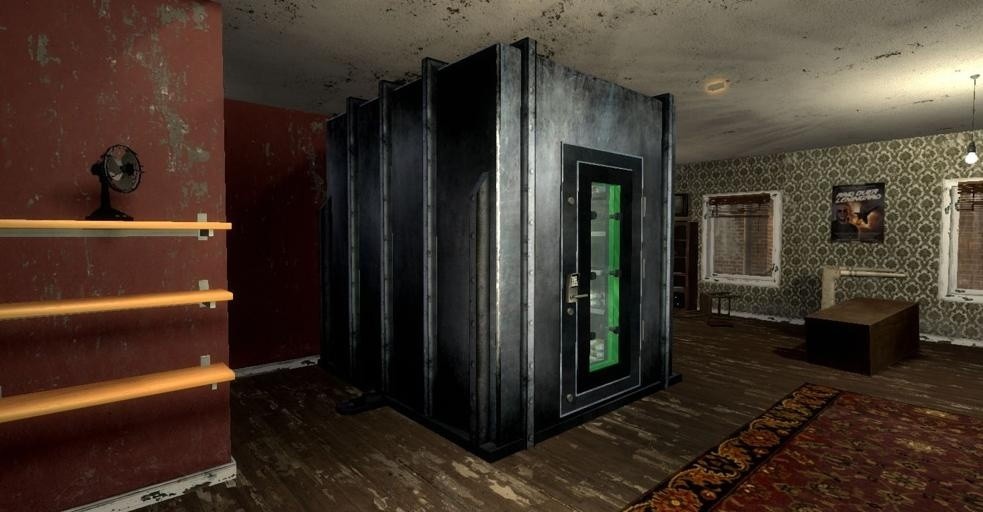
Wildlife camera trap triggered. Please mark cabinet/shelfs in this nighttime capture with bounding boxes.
[671,219,698,312]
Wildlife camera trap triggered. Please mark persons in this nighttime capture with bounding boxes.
[830,204,858,241]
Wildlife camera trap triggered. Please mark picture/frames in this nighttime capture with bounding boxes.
[673,192,688,217]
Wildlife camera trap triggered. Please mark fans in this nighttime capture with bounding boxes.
[81,141,143,222]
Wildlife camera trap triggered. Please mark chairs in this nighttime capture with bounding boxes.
[670,291,741,323]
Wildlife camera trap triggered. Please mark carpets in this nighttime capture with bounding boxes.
[616,381,983,511]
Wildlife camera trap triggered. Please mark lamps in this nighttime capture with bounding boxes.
[963,72,980,165]
